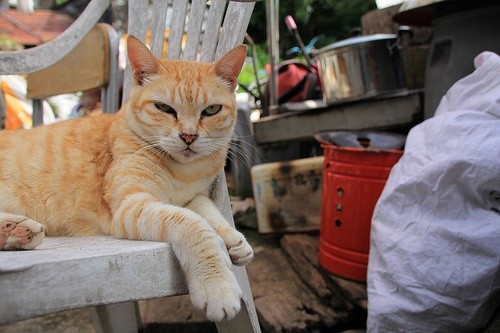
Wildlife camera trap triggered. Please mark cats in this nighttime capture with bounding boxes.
[0,34,254,321]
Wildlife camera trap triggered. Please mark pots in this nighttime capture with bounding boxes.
[312,28,407,107]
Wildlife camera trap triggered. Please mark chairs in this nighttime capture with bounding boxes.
[26,22,119,128]
[0,0,262,333]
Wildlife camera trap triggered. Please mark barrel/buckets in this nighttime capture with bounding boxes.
[318,143,404,283]
